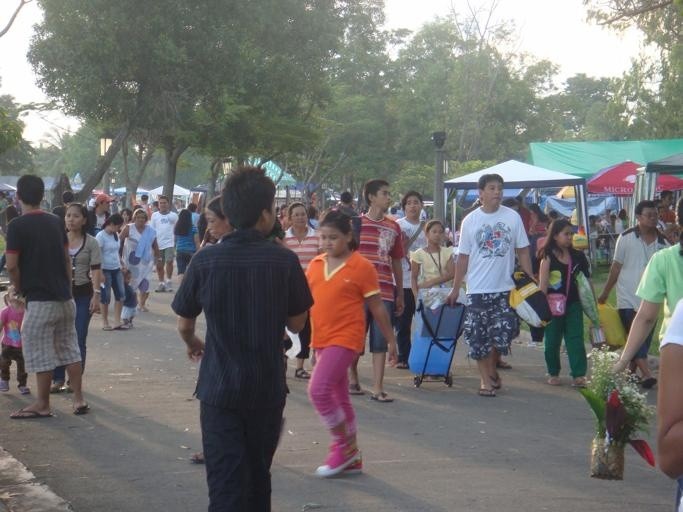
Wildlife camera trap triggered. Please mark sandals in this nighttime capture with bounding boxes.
[295,368,311,378]
[371,391,393,402]
[189,450,204,462]
[497,361,512,369]
[348,384,364,395]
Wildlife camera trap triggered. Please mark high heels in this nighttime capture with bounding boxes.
[50,382,63,393]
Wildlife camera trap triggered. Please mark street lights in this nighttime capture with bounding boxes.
[98,133,115,194]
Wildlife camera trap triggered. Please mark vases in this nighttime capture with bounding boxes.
[590,434,624,482]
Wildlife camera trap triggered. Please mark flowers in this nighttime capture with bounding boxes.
[574,344,656,470]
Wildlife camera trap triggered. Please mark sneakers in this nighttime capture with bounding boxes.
[18,385,30,394]
[155,283,165,292]
[548,377,560,385]
[571,377,588,388]
[0,378,9,391]
[342,457,362,473]
[164,280,173,291]
[316,433,361,477]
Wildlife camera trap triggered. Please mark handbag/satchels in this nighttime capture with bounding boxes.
[545,252,572,316]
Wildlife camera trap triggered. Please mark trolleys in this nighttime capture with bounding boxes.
[407,301,466,388]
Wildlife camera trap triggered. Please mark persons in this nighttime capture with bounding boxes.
[0,204,19,273]
[306,211,398,479]
[589,215,602,233]
[347,180,404,402]
[337,192,358,217]
[599,201,673,388]
[394,191,429,369]
[187,202,200,225]
[445,174,534,398]
[150,196,179,292]
[411,220,455,300]
[658,298,683,507]
[615,209,628,234]
[95,213,129,331]
[53,206,67,230]
[118,209,161,312]
[279,205,288,225]
[539,219,598,387]
[5,174,90,418]
[141,195,149,208]
[658,191,675,221]
[117,208,132,235]
[49,204,101,396]
[191,195,231,464]
[170,167,315,512]
[529,204,548,274]
[0,192,8,208]
[280,204,319,379]
[609,196,683,373]
[173,209,201,289]
[0,284,30,394]
[151,201,159,214]
[62,190,75,207]
[93,194,110,230]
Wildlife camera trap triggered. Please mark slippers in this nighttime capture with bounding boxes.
[112,324,129,330]
[102,326,112,331]
[479,386,496,397]
[10,407,53,418]
[73,400,88,414]
[489,372,501,389]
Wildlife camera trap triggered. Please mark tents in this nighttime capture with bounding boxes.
[147,183,190,198]
[113,187,148,195]
[445,160,593,269]
[583,159,679,199]
[645,153,683,202]
